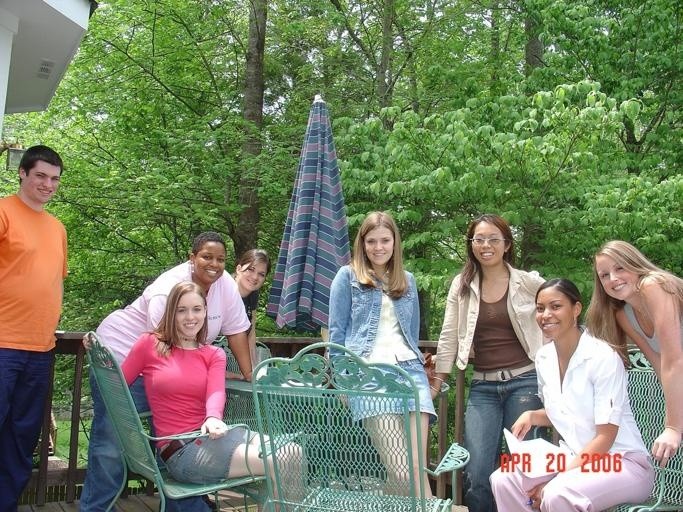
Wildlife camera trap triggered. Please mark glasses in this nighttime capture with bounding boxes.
[470,235,508,245]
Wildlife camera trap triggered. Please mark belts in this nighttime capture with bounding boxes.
[472,362,535,382]
[161,438,196,462]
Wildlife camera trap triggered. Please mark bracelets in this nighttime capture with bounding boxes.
[430,384,442,394]
[432,374,447,385]
[664,422,682,436]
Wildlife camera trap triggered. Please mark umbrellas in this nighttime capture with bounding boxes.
[267,94,351,335]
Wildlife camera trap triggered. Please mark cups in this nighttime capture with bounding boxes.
[253,347,271,380]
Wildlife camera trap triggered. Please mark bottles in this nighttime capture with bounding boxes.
[321,349,337,397]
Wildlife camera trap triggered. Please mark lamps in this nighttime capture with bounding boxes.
[0,134,26,171]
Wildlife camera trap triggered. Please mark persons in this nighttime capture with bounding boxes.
[326,209,440,511]
[586,239,683,468]
[488,278,658,511]
[83,282,305,511]
[77,232,256,510]
[231,249,271,367]
[430,215,546,511]
[0,146,68,511]
